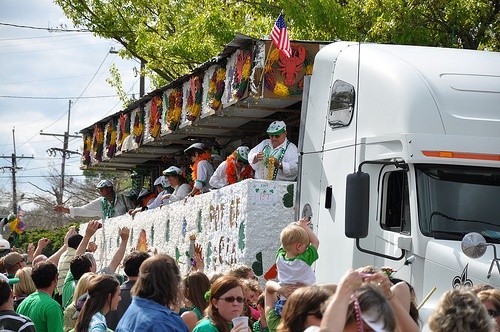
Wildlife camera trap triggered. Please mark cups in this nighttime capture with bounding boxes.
[232,316,248,332]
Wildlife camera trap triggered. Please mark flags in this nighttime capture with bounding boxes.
[269,11,293,57]
[17,206,24,217]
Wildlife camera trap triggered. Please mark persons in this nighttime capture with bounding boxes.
[53,180,136,219]
[126,189,158,215]
[247,120,300,181]
[159,165,193,203]
[0,209,499,332]
[145,174,174,208]
[210,144,253,187]
[183,142,217,198]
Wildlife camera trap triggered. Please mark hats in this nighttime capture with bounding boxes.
[96,179,114,189]
[183,143,207,154]
[153,176,162,188]
[160,174,172,188]
[0,252,28,269]
[162,166,184,176]
[0,240,11,251]
[135,189,151,200]
[124,189,140,197]
[0,272,19,288]
[236,145,250,163]
[266,120,286,136]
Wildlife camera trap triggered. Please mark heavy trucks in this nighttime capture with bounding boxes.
[75,35,499,332]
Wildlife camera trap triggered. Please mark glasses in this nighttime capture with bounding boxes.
[56,271,60,278]
[305,311,330,320]
[215,296,248,304]
[486,308,500,318]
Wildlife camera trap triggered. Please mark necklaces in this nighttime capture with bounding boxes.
[264,141,289,179]
[188,152,209,178]
[225,154,251,184]
[101,192,116,217]
